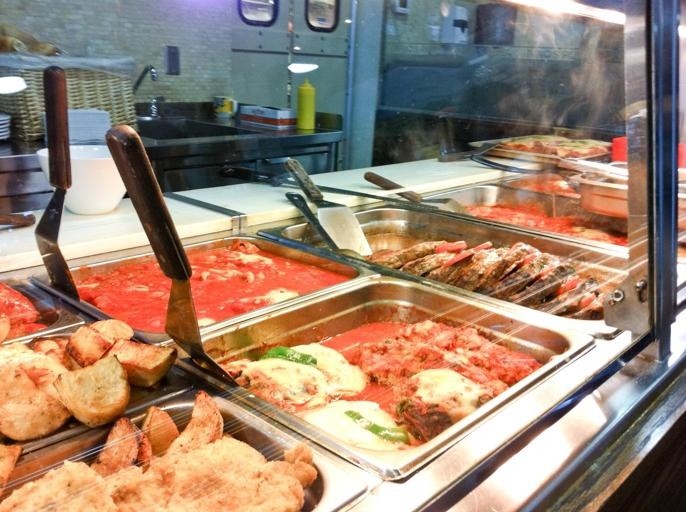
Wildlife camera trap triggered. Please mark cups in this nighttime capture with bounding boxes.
[211,95,238,119]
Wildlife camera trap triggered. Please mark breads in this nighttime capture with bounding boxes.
[0,22,63,56]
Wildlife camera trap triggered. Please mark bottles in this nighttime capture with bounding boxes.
[297,77,317,130]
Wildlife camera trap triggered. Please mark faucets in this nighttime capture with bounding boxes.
[132,62,160,95]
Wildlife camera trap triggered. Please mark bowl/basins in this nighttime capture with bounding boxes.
[36,144,128,215]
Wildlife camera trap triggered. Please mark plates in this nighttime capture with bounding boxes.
[0,112,12,140]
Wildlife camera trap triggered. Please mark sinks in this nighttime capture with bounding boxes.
[133,113,262,141]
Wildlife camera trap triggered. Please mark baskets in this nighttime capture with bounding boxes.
[1,65,139,143]
